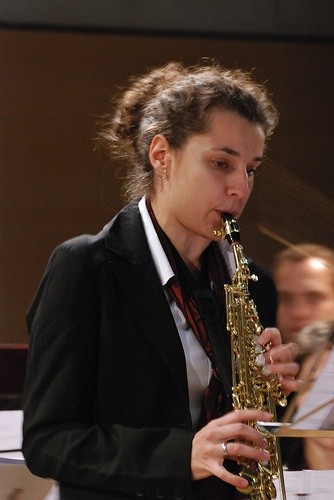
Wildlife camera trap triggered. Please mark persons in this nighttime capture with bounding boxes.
[271,242,334,471]
[19,52,306,499]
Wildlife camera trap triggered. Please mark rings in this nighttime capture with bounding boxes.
[220,442,230,458]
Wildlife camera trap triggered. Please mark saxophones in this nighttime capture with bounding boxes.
[208,212,290,499]
